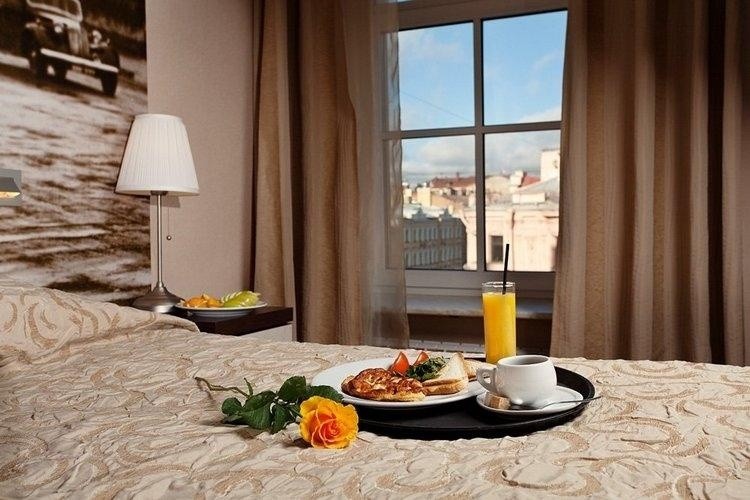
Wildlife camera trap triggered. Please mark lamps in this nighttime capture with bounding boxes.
[114,113,201,315]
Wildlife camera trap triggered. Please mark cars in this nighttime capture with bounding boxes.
[20,0,120,95]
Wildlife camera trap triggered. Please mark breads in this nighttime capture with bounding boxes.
[424,352,469,394]
[342,367,425,401]
[463,356,494,381]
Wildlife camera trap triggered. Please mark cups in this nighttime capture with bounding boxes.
[478,355,560,407]
[482,280,516,364]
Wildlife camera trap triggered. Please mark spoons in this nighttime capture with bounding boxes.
[511,395,603,410]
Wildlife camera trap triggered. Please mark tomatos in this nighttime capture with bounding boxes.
[390,351,429,374]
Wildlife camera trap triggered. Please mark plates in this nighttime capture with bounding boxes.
[310,356,496,410]
[177,296,271,319]
[476,384,585,417]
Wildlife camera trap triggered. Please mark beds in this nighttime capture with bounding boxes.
[0,287,750,500]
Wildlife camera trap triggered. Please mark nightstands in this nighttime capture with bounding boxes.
[113,295,297,340]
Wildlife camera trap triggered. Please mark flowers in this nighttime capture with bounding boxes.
[195,377,359,451]
